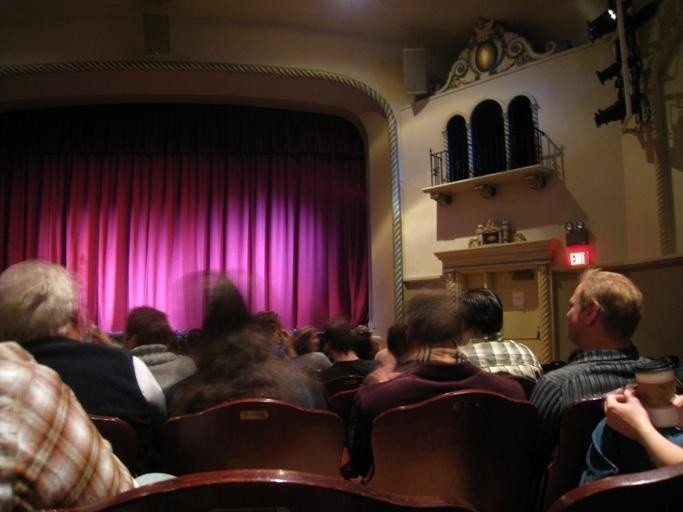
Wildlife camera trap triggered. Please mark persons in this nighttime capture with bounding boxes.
[0,261,169,439]
[0,339,179,512]
[345,288,526,458]
[579,393,683,487]
[529,267,682,473]
[122,275,416,482]
[456,286,544,384]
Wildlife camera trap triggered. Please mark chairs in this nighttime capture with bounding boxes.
[32,372,683,512]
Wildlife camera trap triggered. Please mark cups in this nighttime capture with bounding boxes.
[632,359,680,428]
